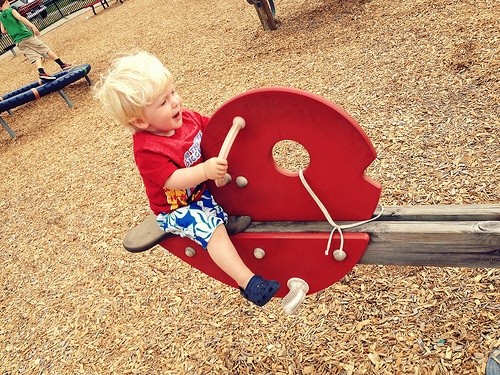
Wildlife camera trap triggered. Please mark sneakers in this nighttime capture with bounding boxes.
[61,63,72,70]
[39,74,56,79]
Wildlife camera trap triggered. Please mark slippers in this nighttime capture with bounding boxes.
[239,275,280,306]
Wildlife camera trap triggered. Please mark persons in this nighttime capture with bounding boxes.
[0,0,73,81]
[94,48,280,306]
